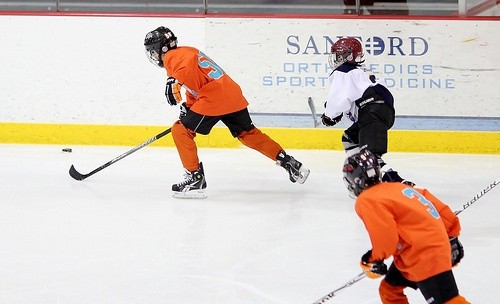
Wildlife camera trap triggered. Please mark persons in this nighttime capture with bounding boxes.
[322,38,416,186]
[343,149,471,304]
[144,26,310,198]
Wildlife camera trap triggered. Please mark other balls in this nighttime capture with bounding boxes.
[62,148,72,152]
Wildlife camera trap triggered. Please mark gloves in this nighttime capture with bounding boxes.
[179,102,190,121]
[321,112,343,127]
[165,77,183,106]
[449,237,464,267]
[360,250,387,278]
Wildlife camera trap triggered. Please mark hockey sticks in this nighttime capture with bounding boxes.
[69,128,171,180]
[313,271,366,304]
[454,180,500,214]
[308,96,324,126]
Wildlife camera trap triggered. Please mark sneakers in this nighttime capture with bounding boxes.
[276,150,310,184]
[401,179,416,188]
[172,162,207,199]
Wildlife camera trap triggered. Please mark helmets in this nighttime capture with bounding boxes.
[342,150,379,200]
[144,26,178,69]
[328,37,364,70]
